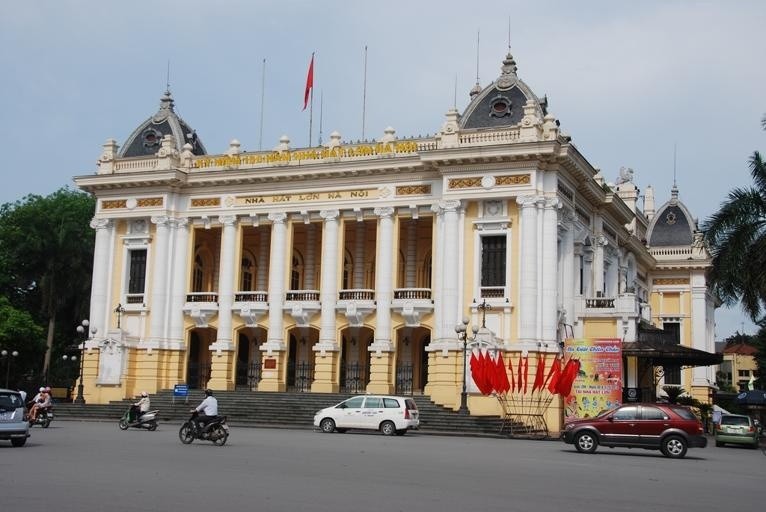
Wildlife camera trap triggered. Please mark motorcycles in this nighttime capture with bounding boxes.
[177,411,231,447]
[117,403,161,433]
[25,399,55,430]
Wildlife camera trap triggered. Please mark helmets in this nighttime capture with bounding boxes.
[40,387,50,391]
[205,389,213,396]
[142,392,148,396]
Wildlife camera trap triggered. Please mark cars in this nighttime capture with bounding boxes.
[0,387,32,449]
[558,401,710,460]
[715,413,760,450]
[753,419,760,426]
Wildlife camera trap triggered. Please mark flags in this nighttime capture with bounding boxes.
[469,351,580,397]
[301,59,313,112]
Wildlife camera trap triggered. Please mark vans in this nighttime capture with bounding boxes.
[309,393,424,437]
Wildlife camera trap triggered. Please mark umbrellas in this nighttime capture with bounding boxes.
[733,389,766,407]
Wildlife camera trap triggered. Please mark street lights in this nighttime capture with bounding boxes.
[0,348,20,388]
[74,319,98,403]
[476,299,492,327]
[453,315,476,418]
[113,303,126,328]
[60,355,78,402]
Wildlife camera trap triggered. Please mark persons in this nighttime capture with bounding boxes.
[189,389,218,435]
[10,386,52,420]
[131,392,151,423]
